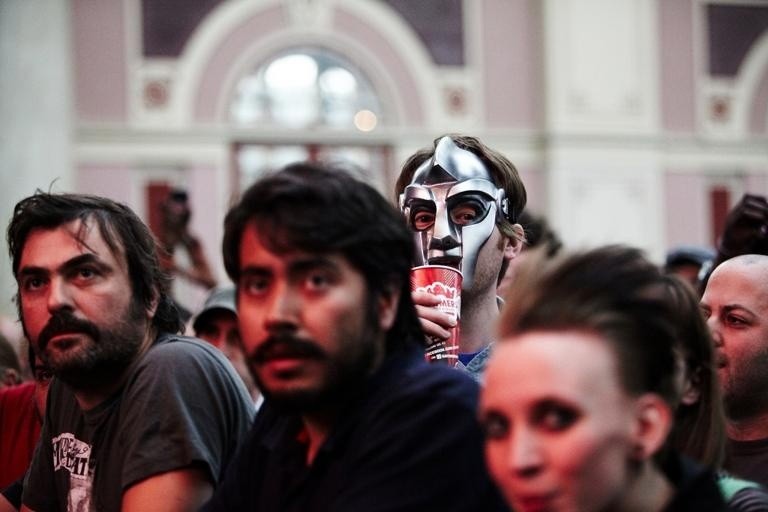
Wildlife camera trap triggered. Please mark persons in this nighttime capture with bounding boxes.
[663,194,768,511]
[1,334,53,511]
[475,243,733,512]
[395,135,563,389]
[8,177,258,511]
[193,288,265,414]
[211,160,513,512]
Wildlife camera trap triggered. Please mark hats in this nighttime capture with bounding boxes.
[195,288,237,333]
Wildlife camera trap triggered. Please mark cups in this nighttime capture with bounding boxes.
[409,264,464,370]
